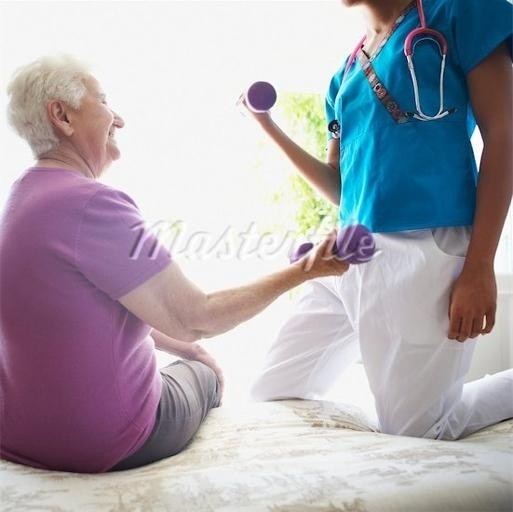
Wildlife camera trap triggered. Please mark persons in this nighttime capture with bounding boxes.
[1,49,359,473]
[233,0,512,444]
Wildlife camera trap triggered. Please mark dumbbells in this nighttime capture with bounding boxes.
[290,224,375,265]
